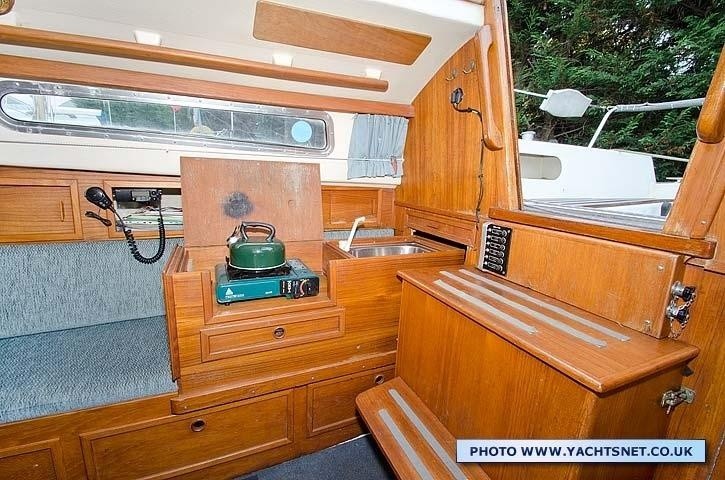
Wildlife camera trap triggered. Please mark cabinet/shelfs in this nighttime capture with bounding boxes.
[304,362,395,440]
[0,178,84,245]
[77,388,296,480]
[198,305,344,365]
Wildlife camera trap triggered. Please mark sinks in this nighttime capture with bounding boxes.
[336,241,441,258]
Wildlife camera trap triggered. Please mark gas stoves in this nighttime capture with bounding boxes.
[213,257,321,306]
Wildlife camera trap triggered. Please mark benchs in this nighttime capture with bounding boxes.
[353,377,494,480]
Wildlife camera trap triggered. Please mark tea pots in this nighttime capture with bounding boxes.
[225,221,284,270]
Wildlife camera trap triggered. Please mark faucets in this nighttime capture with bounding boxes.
[339,216,365,252]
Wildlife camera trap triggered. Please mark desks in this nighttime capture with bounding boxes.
[392,261,703,480]
